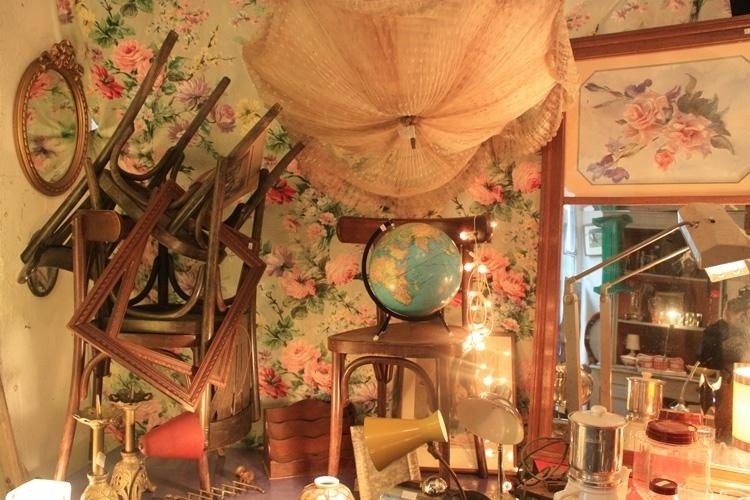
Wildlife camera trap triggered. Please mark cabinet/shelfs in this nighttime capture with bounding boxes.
[583,224,725,422]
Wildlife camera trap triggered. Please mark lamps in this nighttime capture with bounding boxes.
[450,383,552,500]
[618,333,641,367]
[363,411,471,500]
[552,196,748,500]
[594,239,750,420]
[728,358,748,455]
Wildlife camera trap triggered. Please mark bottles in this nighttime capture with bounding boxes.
[631,420,711,500]
[298,474,353,500]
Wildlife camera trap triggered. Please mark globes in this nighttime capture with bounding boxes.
[362,220,463,341]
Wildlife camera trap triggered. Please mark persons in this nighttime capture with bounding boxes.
[699,299,750,364]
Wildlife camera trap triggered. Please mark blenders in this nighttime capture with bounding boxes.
[553,403,629,500]
[623,371,663,447]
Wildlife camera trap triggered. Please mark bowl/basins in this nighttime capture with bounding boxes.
[684,365,718,378]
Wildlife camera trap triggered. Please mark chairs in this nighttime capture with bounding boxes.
[320,205,504,496]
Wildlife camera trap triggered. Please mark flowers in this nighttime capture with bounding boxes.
[573,62,744,186]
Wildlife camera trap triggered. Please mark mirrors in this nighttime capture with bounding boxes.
[500,6,749,498]
